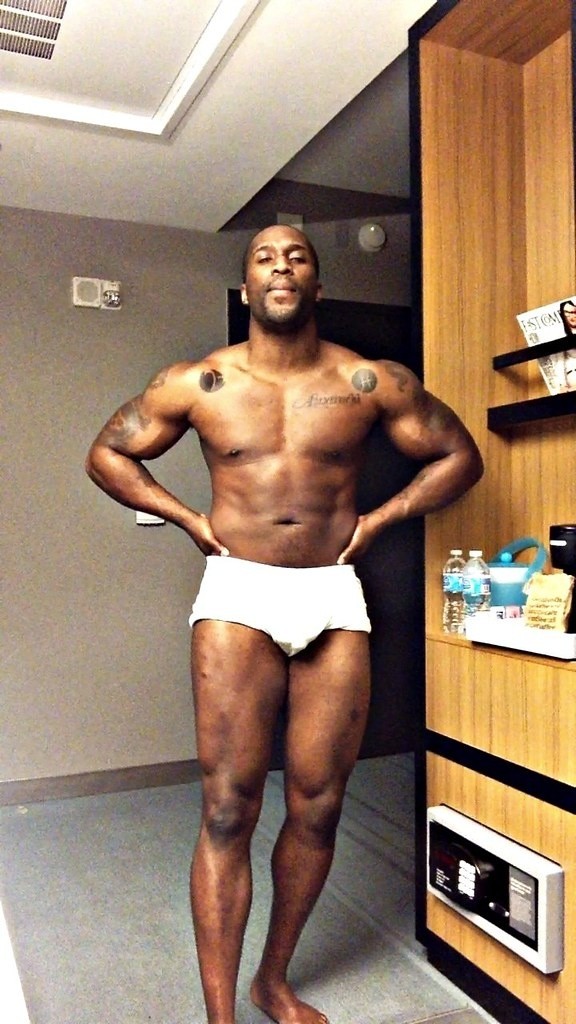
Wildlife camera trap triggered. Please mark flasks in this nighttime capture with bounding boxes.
[549,524,576,633]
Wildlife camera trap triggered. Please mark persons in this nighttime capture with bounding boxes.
[85,222,485,1024]
[561,301,576,394]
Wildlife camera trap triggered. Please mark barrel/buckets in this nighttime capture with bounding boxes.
[484,537,545,614]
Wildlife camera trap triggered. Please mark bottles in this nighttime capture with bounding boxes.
[441,549,468,634]
[461,549,492,619]
